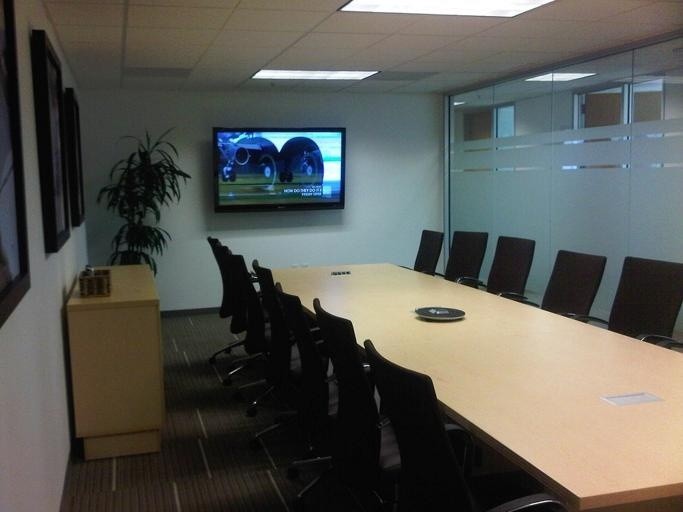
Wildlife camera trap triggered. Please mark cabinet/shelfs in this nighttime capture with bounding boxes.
[60,262,168,461]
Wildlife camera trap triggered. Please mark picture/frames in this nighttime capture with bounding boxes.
[28,26,72,257]
[0,0,32,332]
[64,85,87,230]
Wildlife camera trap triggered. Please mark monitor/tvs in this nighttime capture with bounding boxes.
[212,126,345,213]
